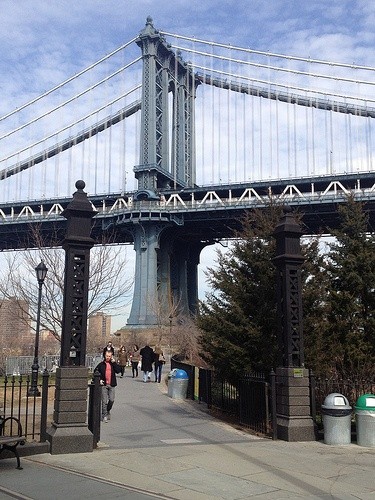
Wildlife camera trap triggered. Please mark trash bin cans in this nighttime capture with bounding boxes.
[172,370,189,399]
[168,368,179,397]
[321,393,353,445]
[354,394,375,447]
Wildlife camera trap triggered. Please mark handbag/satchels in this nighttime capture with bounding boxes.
[158,353,166,362]
[137,361,142,368]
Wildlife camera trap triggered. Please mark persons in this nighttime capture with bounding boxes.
[103,341,114,361]
[131,344,140,378]
[140,341,156,383]
[94,349,122,421]
[118,346,129,378]
[154,344,165,384]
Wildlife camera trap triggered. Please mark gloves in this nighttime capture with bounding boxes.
[111,358,116,363]
[100,380,105,385]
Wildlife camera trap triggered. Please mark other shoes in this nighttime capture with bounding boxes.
[108,414,110,419]
[103,417,108,422]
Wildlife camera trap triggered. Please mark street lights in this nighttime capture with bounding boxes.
[26,258,49,397]
[168,314,174,357]
